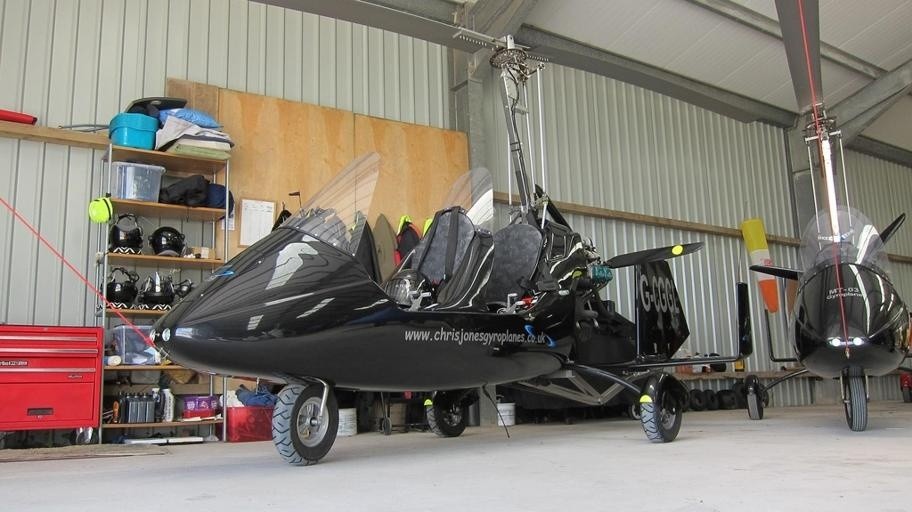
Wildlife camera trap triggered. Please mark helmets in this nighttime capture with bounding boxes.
[147,227,185,256]
[101,282,138,304]
[109,226,144,248]
[137,271,175,304]
[385,268,435,306]
[89,197,113,223]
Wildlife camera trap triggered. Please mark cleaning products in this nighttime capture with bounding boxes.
[112,388,176,423]
[693,352,711,374]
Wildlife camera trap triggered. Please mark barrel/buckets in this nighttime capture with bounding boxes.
[498,401,516,427]
[376,398,408,432]
[338,406,358,437]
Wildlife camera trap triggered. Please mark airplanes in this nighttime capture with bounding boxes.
[745,97,912,433]
[149,25,755,467]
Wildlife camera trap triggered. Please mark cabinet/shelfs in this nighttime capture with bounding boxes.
[94,143,229,444]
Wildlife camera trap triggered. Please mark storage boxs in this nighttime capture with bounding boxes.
[109,113,159,150]
[186,246,215,260]
[108,325,155,365]
[111,161,167,202]
[215,406,273,442]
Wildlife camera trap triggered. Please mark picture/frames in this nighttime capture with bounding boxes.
[238,197,277,248]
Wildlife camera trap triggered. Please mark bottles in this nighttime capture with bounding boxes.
[691,352,712,374]
[735,359,744,372]
[113,386,175,423]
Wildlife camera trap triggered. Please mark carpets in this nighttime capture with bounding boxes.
[0,444,167,462]
